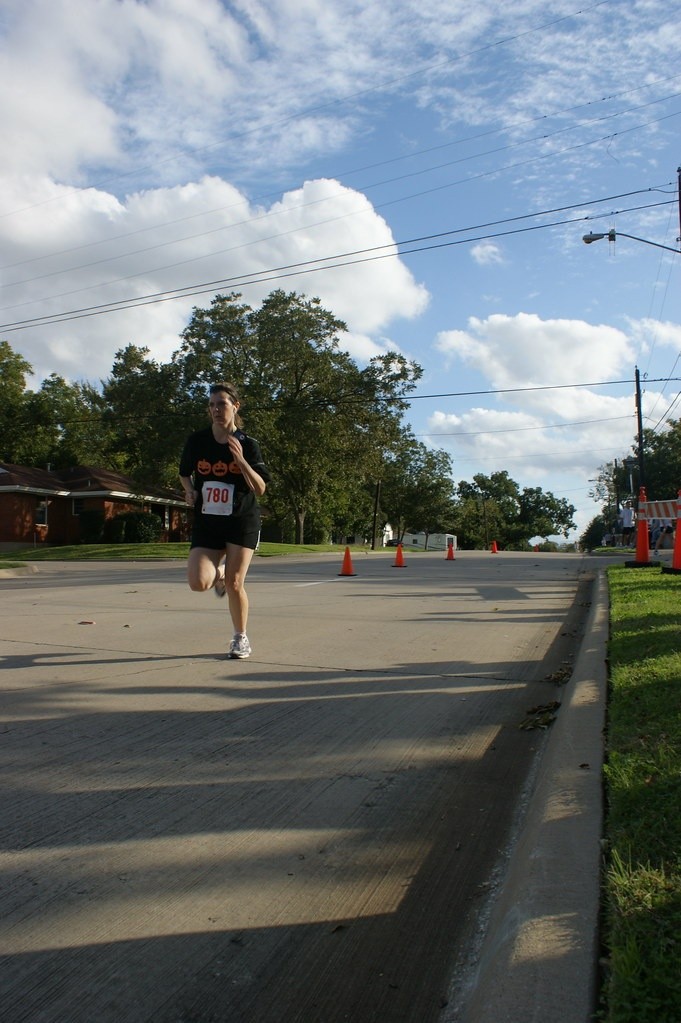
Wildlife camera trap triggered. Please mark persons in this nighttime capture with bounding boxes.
[605,531,613,547]
[178,381,271,658]
[618,500,637,547]
[650,518,675,555]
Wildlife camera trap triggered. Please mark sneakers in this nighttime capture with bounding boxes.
[227,634,252,658]
[215,564,226,598]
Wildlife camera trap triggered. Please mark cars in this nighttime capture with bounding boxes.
[385,540,404,547]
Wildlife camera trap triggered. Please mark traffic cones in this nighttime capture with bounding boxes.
[337,545,358,576]
[625,486,661,567]
[392,544,407,567]
[491,541,498,554]
[661,488,681,574]
[445,544,456,560]
